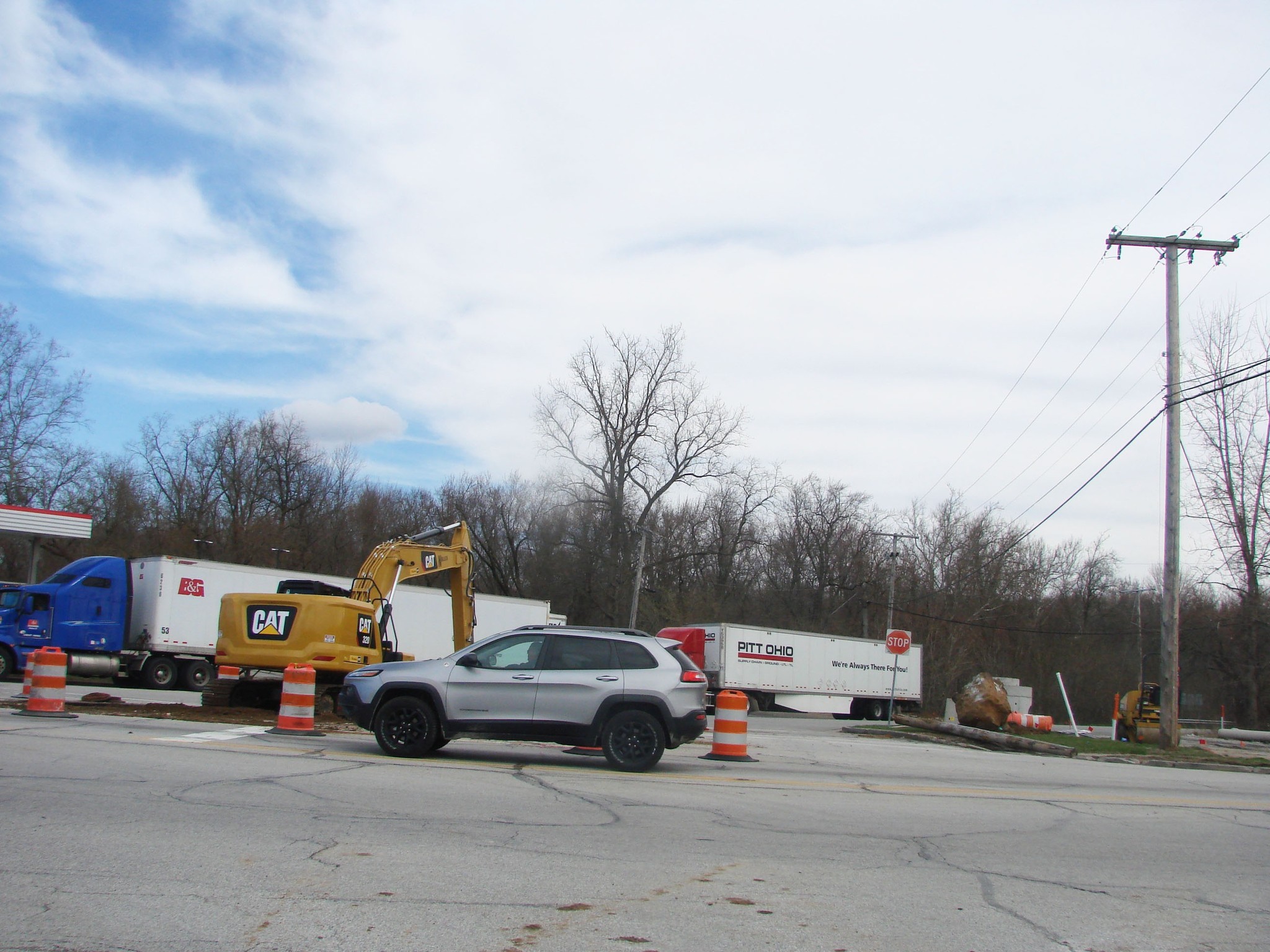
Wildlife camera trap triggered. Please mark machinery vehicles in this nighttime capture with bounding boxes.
[213,522,479,718]
[1116,682,1183,748]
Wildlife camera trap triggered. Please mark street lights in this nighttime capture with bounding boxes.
[271,548,290,568]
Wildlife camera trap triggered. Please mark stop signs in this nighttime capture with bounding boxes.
[887,630,911,655]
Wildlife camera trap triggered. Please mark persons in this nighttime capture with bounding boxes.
[505,642,543,669]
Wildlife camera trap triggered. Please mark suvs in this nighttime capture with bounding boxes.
[338,624,710,774]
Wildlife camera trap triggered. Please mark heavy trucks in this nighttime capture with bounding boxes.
[0,556,571,690]
[656,622,923,720]
